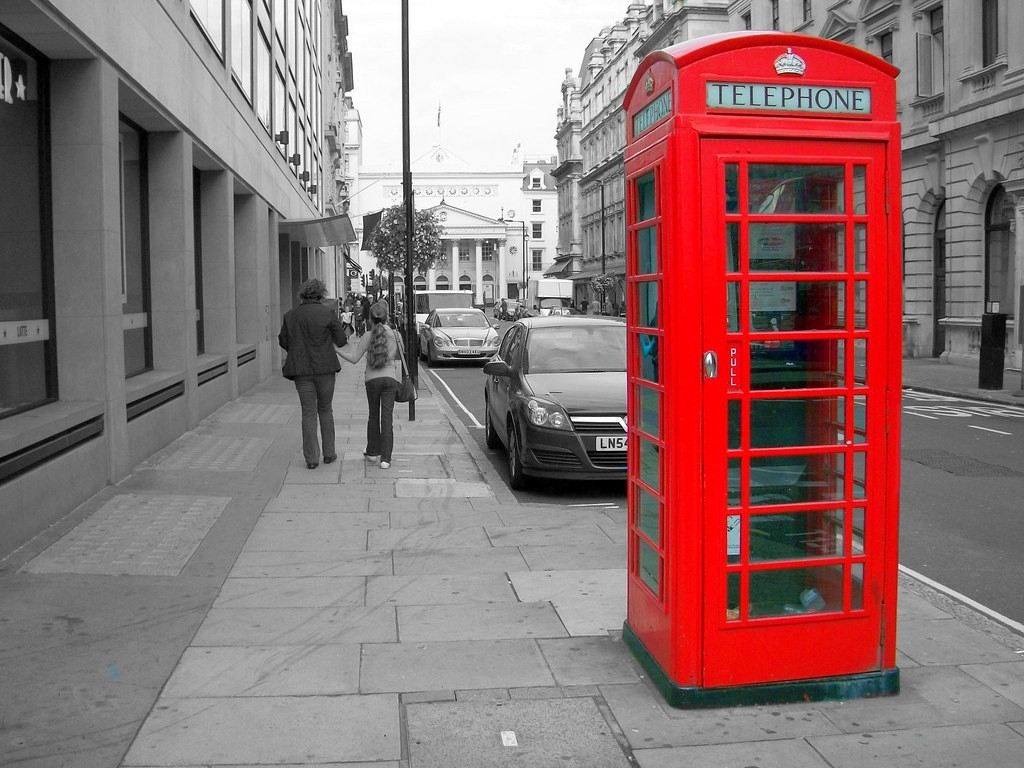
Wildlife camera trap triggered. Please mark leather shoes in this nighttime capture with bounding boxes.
[324,454,337,463]
[307,463,319,469]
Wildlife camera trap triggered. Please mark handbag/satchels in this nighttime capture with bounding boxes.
[394,375,418,402]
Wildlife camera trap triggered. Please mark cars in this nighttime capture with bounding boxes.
[418,308,501,366]
[548,305,572,317]
[483,316,627,494]
[492,297,528,323]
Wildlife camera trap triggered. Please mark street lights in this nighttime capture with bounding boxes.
[525,234,529,295]
[575,173,606,309]
[505,217,525,300]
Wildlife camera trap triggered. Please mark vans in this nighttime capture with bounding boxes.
[414,290,473,346]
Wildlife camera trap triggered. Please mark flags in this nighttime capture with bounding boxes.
[359,211,383,251]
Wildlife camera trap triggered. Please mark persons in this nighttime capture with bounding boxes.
[604,297,613,314]
[501,298,507,319]
[338,292,378,339]
[590,298,601,315]
[581,298,589,314]
[334,303,404,470]
[277,278,347,470]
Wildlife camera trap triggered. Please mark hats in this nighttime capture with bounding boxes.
[367,294,373,299]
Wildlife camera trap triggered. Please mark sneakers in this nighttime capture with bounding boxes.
[366,455,378,462]
[380,461,390,469]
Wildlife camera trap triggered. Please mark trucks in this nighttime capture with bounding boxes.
[527,277,574,315]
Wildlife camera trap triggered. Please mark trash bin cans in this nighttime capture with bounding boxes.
[979,312,1009,391]
